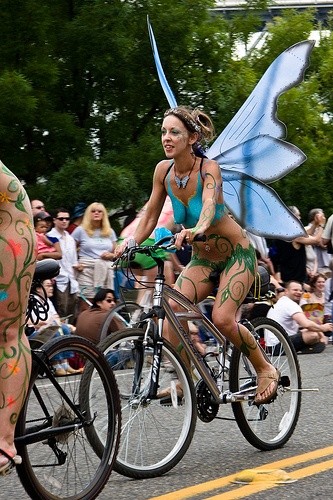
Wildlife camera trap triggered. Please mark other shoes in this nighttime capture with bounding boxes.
[54,363,81,376]
[163,360,194,372]
[124,360,135,369]
[302,341,325,354]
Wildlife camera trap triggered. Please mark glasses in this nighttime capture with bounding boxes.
[31,206,46,210]
[104,298,117,303]
[55,218,71,221]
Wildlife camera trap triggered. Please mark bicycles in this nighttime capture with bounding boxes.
[78,234,319,479]
[14,258,122,500]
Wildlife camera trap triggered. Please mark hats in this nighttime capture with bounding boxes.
[34,211,51,223]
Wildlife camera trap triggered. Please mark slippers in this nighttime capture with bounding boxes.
[254,367,281,404]
[0,449,22,478]
[155,380,185,399]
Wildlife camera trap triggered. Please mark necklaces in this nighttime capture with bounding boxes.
[175,157,197,189]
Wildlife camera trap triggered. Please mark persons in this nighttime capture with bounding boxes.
[1,159,38,473]
[28,200,333,379]
[112,109,280,404]
